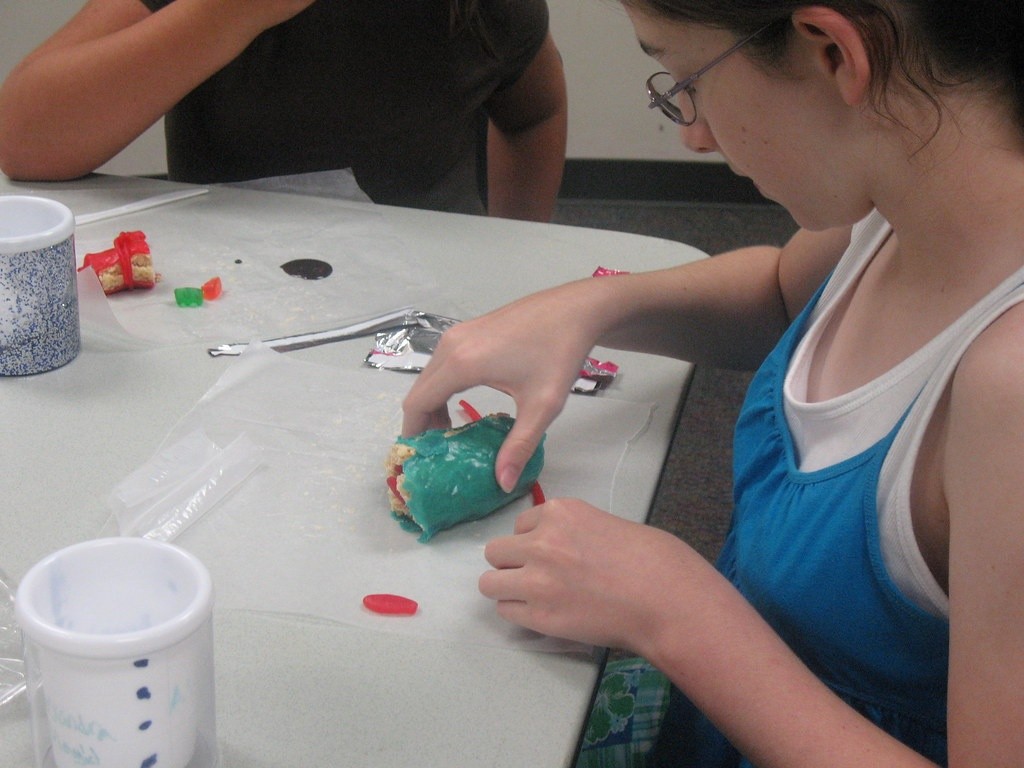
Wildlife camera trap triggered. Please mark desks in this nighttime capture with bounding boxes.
[0,172,715,768]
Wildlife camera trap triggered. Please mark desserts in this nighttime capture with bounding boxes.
[78,230,155,296]
[387,412,546,541]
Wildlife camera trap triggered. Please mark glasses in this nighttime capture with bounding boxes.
[646,8,796,126]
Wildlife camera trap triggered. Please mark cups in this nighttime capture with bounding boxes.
[0,194,81,377]
[16,533,217,764]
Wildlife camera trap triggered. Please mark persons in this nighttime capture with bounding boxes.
[401,0,1024,768]
[0,0,566,225]
